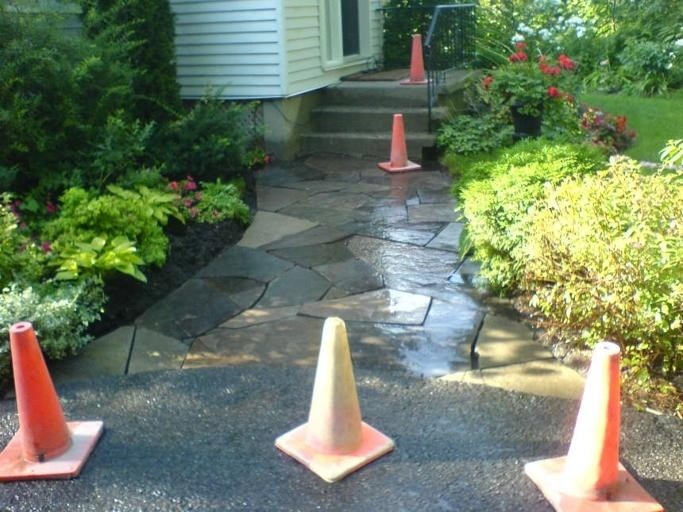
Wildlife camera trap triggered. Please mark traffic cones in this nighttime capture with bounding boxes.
[398,30,433,85]
[520,340,665,512]
[377,111,421,172]
[0,321,104,483]
[271,316,394,485]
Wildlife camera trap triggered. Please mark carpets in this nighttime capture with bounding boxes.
[340,70,409,81]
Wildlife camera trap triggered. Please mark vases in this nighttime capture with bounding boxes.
[508,90,545,146]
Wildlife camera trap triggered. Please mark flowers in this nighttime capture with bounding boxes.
[476,32,578,120]
